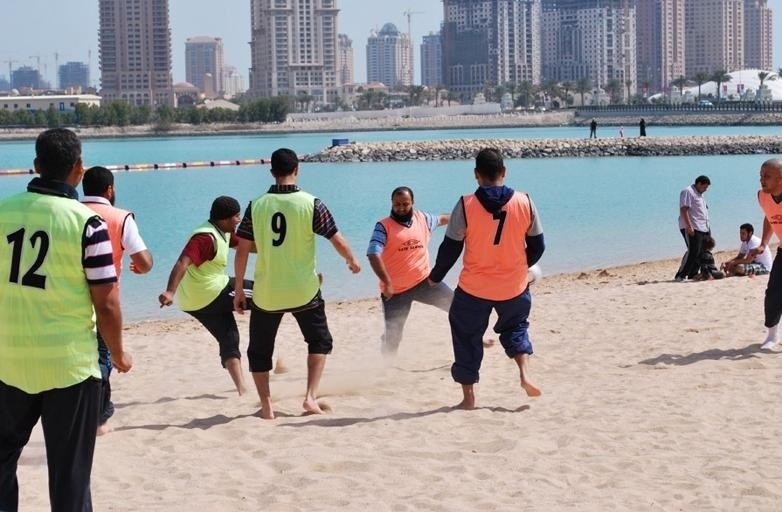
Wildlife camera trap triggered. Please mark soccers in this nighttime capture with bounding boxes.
[526,265,543,285]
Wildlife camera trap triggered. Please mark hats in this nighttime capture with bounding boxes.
[210,196,240,220]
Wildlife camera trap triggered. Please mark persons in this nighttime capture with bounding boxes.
[589,119,597,138]
[159,194,324,392]
[675,158,781,351]
[81,166,153,427]
[639,118,646,136]
[426,147,545,410]
[366,186,496,357]
[0,128,133,512]
[618,126,624,137]
[232,148,361,418]
